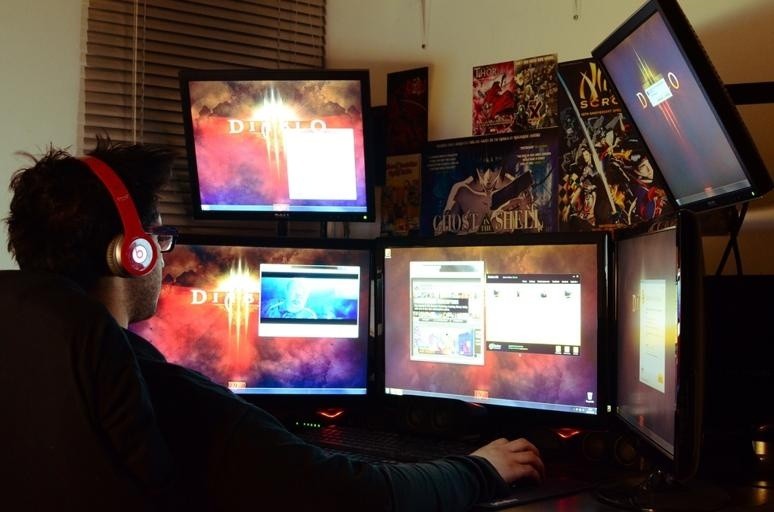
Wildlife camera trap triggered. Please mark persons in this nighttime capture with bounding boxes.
[0,133,544,509]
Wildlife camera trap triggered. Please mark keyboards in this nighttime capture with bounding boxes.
[287,420,405,460]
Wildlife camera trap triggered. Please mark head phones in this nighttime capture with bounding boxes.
[63,153,158,278]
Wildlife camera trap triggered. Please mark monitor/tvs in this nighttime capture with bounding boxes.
[376,230,616,437]
[591,0,770,214]
[128,234,378,410]
[608,225,707,473]
[176,68,376,237]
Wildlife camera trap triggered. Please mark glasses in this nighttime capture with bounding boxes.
[155,230,174,252]
